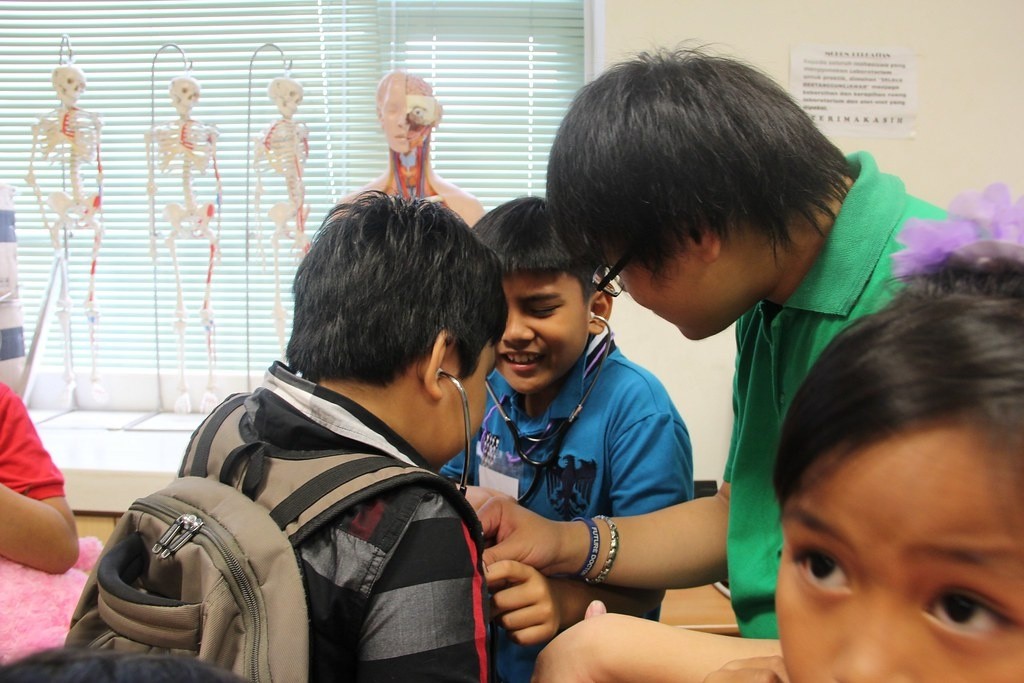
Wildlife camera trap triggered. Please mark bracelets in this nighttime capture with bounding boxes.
[567,514,601,581]
[584,508,620,585]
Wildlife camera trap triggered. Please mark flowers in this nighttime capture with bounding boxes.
[889,183,1024,284]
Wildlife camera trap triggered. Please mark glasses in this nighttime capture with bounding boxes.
[592,242,646,296]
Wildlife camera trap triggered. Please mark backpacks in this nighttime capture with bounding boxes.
[65,392,484,683]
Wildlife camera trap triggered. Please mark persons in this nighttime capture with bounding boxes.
[0,186,1024,683]
[444,44,957,683]
